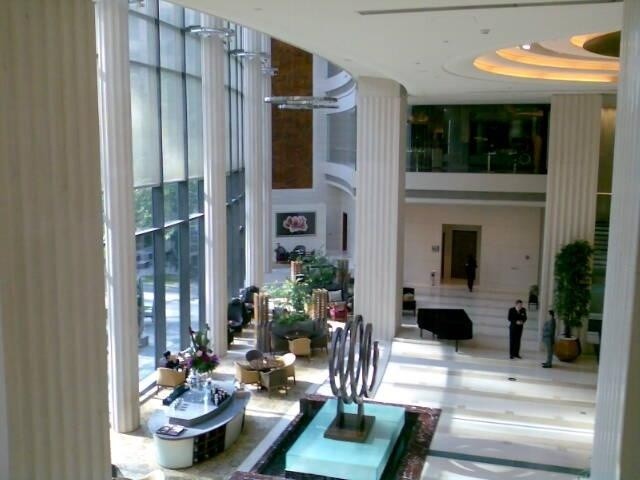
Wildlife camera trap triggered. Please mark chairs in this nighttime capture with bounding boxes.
[270,302,347,364]
[235,350,297,396]
[403,289,416,316]
[229,286,258,342]
[155,367,186,396]
[276,245,315,264]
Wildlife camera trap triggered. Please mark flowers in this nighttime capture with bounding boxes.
[188,323,218,375]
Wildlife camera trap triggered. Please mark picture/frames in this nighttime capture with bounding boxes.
[343,212,348,250]
[277,211,317,238]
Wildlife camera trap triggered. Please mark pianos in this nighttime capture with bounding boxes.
[417,308,473,352]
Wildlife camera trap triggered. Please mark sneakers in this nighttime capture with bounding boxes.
[542,362,553,368]
[510,353,522,359]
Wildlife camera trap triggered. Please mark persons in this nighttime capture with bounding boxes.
[465,260,475,293]
[507,299,528,360]
[158,351,190,384]
[541,309,556,368]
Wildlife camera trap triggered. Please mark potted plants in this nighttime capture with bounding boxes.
[554,239,591,362]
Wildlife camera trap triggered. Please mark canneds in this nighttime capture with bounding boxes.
[211,388,227,406]
